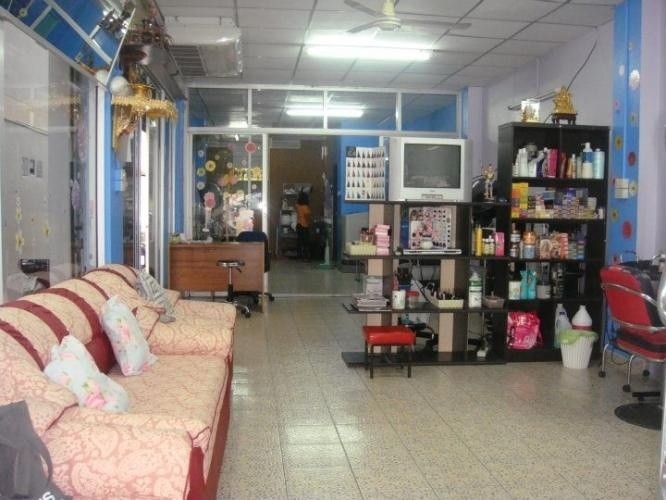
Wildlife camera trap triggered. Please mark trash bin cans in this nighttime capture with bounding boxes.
[560,329,599,369]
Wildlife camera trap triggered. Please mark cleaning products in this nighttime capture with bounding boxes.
[508,266,551,300]
[392,285,411,309]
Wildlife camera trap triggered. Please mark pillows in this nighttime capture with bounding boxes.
[99,295,157,374]
[45,333,129,413]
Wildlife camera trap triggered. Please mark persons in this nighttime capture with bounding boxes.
[294,191,314,263]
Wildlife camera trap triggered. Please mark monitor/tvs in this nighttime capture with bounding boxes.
[383,136,472,202]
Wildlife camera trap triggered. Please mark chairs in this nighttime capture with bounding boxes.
[600,261,666,431]
[228,231,275,304]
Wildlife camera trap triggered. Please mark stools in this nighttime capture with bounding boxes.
[213,259,259,318]
[360,325,416,378]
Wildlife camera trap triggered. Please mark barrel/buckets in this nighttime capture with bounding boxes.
[572,304,593,330]
[555,304,572,348]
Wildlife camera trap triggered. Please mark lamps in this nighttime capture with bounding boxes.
[509,84,564,110]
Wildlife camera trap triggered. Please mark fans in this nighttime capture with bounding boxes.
[341,0,453,36]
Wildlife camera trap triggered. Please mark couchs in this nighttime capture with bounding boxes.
[0,262,237,499]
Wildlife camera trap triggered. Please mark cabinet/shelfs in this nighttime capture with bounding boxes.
[340,123,609,367]
[169,243,265,300]
